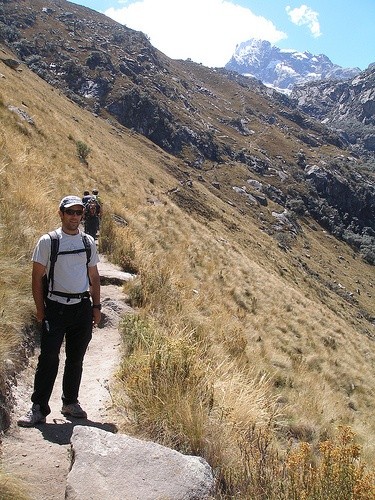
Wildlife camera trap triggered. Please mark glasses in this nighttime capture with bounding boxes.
[66,209,83,215]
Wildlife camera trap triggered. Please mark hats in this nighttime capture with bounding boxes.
[60,195,87,209]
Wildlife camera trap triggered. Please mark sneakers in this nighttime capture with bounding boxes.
[61,402,87,418]
[17,404,46,427]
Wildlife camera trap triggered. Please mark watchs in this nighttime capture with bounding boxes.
[93,304,102,311]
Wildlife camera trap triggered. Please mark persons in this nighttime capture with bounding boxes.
[82,198,103,241]
[82,191,91,205]
[91,189,101,204]
[16,194,101,428]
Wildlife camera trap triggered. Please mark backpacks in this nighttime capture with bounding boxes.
[87,195,99,216]
